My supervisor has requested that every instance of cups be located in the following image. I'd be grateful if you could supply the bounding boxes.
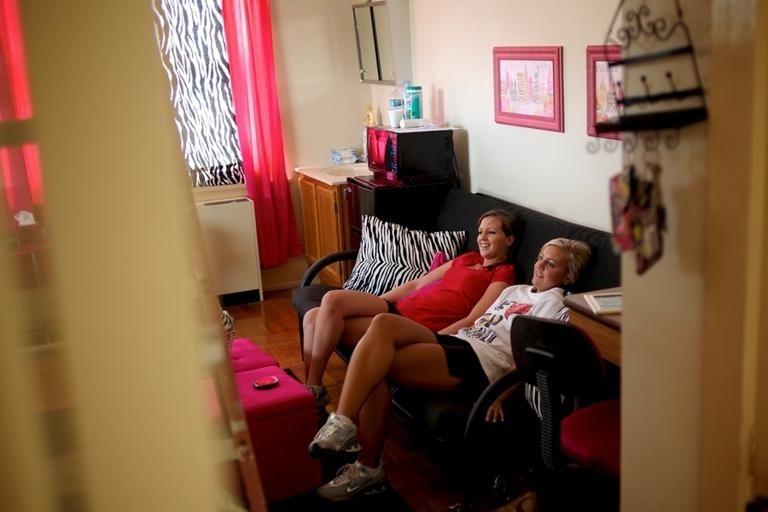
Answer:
[388,97,404,129]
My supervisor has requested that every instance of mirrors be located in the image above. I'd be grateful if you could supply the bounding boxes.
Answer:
[352,1,395,85]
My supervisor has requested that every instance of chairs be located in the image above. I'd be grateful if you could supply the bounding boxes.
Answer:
[511,314,620,484]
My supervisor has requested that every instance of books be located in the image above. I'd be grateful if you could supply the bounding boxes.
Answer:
[583,293,621,316]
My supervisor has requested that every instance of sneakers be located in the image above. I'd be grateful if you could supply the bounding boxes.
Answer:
[308,412,362,458]
[305,383,331,411]
[316,459,389,503]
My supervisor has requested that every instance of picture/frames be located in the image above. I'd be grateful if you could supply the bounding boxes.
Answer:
[586,44,625,140]
[494,46,564,133]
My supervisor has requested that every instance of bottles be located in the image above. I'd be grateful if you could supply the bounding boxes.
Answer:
[405,86,423,128]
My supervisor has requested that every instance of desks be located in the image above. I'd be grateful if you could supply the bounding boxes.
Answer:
[563,282,621,366]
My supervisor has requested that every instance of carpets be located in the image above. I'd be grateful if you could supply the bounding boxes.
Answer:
[265,367,416,512]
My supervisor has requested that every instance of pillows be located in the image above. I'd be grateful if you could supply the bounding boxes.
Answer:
[341,213,468,296]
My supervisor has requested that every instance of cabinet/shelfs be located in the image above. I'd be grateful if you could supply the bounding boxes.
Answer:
[297,173,351,289]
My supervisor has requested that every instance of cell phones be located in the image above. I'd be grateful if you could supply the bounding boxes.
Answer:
[253,375,278,388]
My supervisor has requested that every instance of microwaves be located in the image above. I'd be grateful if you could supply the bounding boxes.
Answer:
[365,126,453,183]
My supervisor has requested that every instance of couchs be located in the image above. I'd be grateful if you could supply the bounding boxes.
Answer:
[289,187,621,512]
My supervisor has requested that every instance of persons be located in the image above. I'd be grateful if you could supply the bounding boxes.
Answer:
[304,238,592,501]
[304,207,514,430]
